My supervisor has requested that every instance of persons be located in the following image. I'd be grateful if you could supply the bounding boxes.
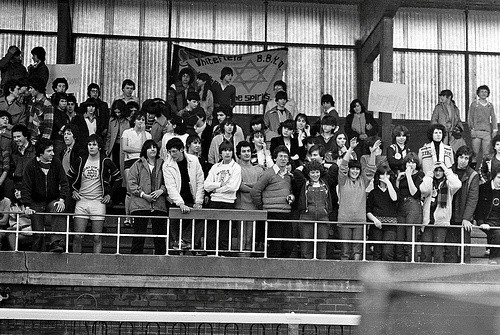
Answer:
[293,157,337,259]
[205,141,242,257]
[162,137,204,251]
[251,145,294,259]
[0,45,500,265]
[127,140,168,255]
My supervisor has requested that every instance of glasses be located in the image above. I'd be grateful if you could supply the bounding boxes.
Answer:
[91,90,100,94]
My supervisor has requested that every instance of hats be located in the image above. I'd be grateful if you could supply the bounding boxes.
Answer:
[439,90,452,95]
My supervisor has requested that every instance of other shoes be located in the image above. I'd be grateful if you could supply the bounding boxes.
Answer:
[50,245,64,253]
[488,260,498,265]
[123,219,131,226]
